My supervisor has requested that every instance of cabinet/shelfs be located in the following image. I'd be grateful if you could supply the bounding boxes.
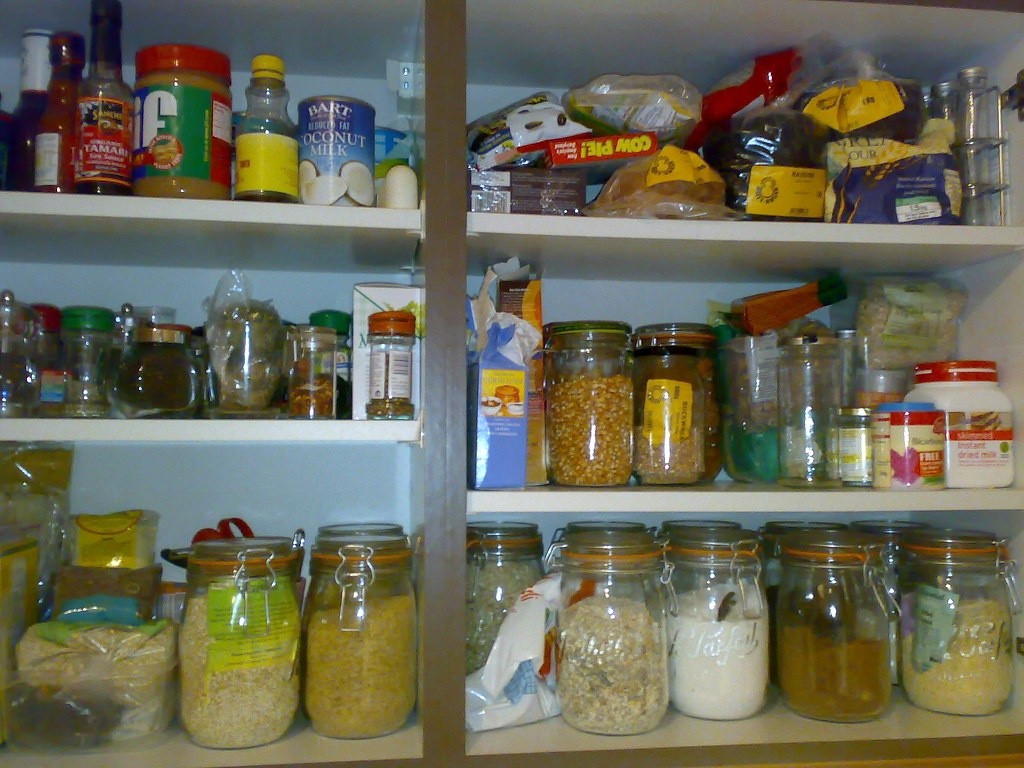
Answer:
[467,207,1024,768]
[2,187,426,768]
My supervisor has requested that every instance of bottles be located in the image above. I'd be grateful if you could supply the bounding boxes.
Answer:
[235,54,299,203]
[776,334,847,488]
[0,0,133,198]
[0,289,416,419]
[178,517,1015,747]
[543,323,723,502]
[923,67,994,227]
